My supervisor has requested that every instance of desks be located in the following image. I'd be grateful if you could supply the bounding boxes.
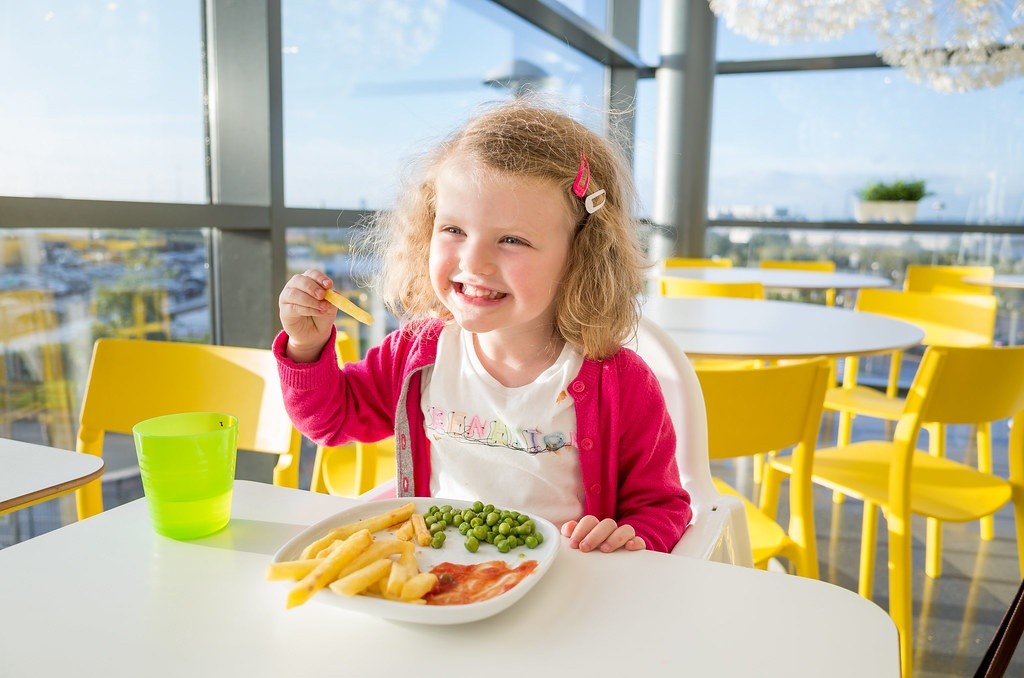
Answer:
[964,274,1024,289]
[646,267,893,289]
[631,295,924,361]
[0,438,903,678]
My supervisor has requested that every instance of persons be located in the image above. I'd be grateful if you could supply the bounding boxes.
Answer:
[273,108,692,554]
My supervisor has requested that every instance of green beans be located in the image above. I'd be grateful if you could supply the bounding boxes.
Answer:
[422,501,544,552]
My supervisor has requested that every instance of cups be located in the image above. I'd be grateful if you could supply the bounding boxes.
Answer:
[132,412,237,540]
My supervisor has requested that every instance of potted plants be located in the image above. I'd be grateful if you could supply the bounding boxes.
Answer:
[849,179,927,225]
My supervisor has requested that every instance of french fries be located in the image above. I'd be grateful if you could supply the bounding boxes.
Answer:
[324,288,374,326]
[267,500,439,610]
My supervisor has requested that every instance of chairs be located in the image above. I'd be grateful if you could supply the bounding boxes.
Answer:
[75,262,1024,678]
[0,234,379,527]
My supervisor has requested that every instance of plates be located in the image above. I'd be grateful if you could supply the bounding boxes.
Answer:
[269,496,561,624]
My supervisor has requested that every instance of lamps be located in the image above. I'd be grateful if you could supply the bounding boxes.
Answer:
[706,0,1024,97]
